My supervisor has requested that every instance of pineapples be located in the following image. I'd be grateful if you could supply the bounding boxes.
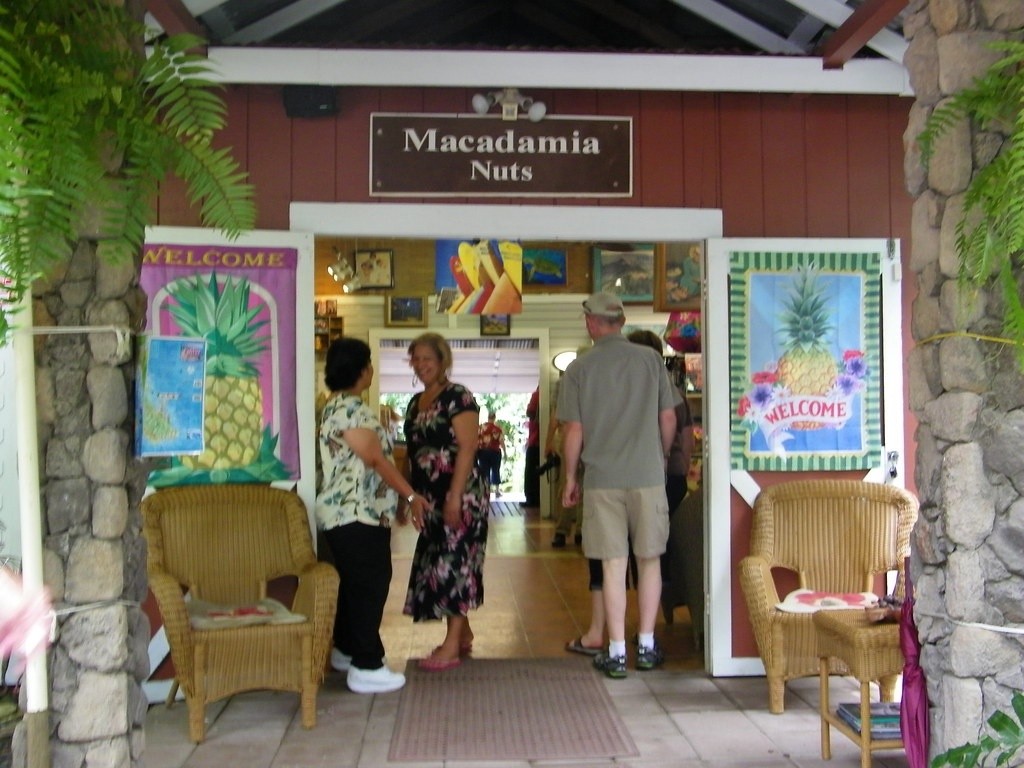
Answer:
[774,271,840,431]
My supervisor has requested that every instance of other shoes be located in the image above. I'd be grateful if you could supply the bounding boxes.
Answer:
[552,533,566,547]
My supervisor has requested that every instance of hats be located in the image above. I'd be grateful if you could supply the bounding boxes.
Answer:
[581,292,623,317]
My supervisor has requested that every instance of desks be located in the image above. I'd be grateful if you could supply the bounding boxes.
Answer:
[815,610,905,768]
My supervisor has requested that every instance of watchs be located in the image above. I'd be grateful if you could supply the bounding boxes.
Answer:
[407,491,418,504]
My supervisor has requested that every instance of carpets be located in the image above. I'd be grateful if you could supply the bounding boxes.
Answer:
[386,654,638,763]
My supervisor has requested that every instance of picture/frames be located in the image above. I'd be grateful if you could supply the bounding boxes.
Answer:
[351,249,393,288]
[524,247,568,284]
[480,314,509,335]
[590,243,702,311]
[386,291,428,325]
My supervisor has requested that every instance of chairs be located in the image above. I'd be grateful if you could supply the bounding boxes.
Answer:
[741,481,918,707]
[141,487,340,741]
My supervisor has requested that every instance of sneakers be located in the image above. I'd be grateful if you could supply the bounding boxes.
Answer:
[347,664,406,693]
[330,647,386,671]
[635,644,664,668]
[594,652,627,678]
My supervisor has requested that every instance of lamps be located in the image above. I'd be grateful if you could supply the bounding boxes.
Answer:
[471,86,548,123]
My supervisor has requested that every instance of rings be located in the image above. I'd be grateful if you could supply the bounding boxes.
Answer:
[412,515,416,523]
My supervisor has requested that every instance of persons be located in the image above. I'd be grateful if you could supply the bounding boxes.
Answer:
[357,253,388,285]
[474,411,507,499]
[565,527,639,653]
[390,332,489,673]
[554,290,684,679]
[551,345,589,549]
[519,381,540,508]
[317,337,433,695]
[626,329,695,646]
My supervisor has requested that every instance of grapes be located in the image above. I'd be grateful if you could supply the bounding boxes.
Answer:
[877,595,905,607]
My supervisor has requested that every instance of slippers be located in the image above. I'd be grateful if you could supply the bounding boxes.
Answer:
[433,631,473,651]
[419,647,461,671]
[566,637,603,655]
